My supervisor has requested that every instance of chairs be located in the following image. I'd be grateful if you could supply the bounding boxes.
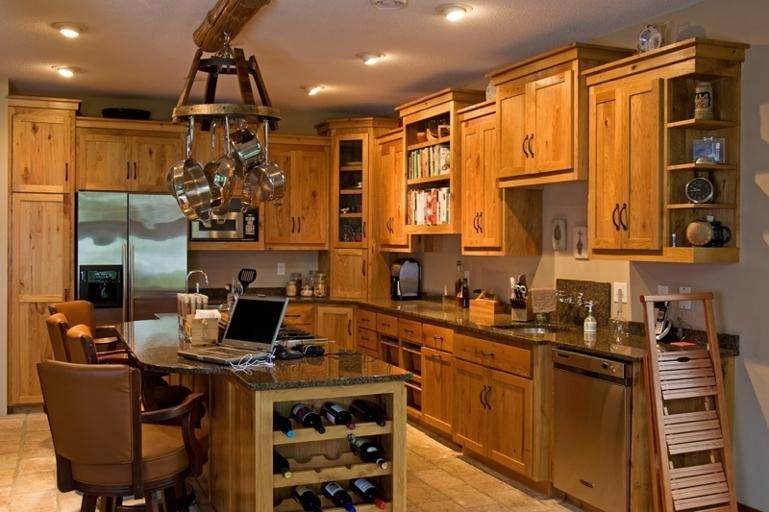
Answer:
[35,358,212,511]
[46,313,126,362]
[66,323,194,511]
[46,301,123,346]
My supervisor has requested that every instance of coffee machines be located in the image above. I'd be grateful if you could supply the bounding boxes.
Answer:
[389,256,422,300]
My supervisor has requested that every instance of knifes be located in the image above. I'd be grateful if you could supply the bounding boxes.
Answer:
[509,272,528,310]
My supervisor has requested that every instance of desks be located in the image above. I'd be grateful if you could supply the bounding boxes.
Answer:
[112,310,357,512]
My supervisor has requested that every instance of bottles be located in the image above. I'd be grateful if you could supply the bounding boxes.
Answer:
[274,400,388,511]
[176,293,210,319]
[612,287,629,352]
[454,259,470,308]
[694,80,713,121]
[286,270,328,298]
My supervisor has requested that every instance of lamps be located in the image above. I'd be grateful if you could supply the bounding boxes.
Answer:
[443,7,465,22]
[305,84,322,96]
[361,54,380,65]
[57,67,80,79]
[59,26,80,39]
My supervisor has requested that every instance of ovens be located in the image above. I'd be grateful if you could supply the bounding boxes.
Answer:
[549,347,631,511]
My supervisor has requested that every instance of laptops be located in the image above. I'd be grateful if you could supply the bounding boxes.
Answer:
[177,293,289,365]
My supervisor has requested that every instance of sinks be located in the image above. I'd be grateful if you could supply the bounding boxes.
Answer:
[488,322,573,335]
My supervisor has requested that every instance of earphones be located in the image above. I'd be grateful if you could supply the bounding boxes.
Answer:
[268,364,273,365]
[264,362,268,364]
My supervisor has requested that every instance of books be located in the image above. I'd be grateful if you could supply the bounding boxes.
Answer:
[406,145,450,225]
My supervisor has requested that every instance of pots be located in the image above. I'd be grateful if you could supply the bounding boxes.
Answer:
[159,114,286,221]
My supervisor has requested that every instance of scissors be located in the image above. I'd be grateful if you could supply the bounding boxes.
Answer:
[515,285,526,298]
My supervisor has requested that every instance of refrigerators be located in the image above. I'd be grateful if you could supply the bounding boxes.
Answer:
[76,191,189,324]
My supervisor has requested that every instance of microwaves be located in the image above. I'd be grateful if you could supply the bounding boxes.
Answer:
[189,207,259,240]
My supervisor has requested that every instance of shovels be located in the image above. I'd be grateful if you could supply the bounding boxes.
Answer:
[238,269,256,295]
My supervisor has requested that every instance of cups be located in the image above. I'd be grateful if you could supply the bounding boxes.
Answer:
[703,220,733,247]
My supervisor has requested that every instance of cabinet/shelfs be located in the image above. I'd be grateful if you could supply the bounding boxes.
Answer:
[376,313,421,413]
[10,96,81,193]
[394,82,485,235]
[452,331,551,480]
[282,304,315,334]
[456,99,542,258]
[374,128,426,253]
[10,194,72,405]
[330,249,397,299]
[225,381,408,512]
[354,308,376,355]
[315,305,354,348]
[331,116,375,248]
[262,135,328,250]
[421,323,452,425]
[591,35,750,264]
[76,117,183,192]
[496,42,634,187]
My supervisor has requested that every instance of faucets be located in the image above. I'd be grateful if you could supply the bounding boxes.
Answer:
[185,269,209,293]
[552,290,573,304]
